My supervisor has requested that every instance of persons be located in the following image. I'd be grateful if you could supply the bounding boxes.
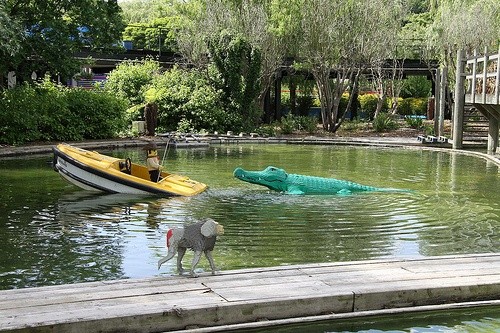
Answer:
[143,148,165,183]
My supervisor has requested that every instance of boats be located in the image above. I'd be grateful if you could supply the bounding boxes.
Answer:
[52,141,211,196]
